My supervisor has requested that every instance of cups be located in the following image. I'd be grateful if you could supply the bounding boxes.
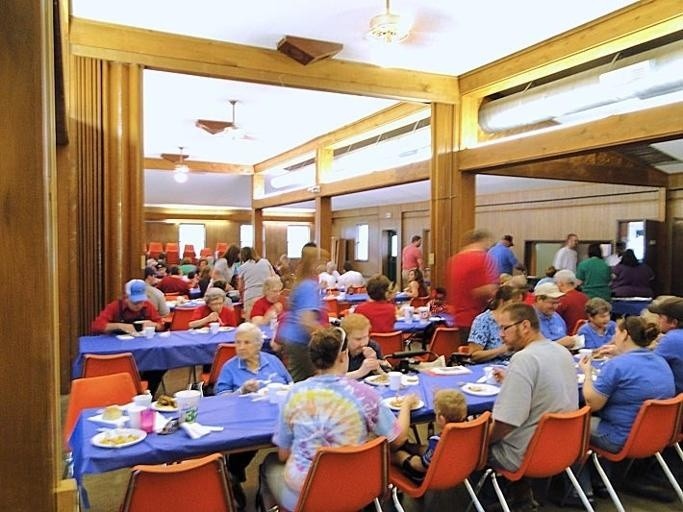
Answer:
[276,390,289,412]
[266,381,282,404]
[133,394,153,409]
[385,371,403,390]
[128,406,147,429]
[174,389,200,426]
[141,408,155,433]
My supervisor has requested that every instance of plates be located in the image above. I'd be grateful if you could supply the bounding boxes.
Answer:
[573,352,610,360]
[87,413,130,426]
[382,395,426,411]
[129,314,446,337]
[88,427,148,448]
[465,382,500,396]
[152,399,181,412]
[364,373,391,385]
[577,372,597,383]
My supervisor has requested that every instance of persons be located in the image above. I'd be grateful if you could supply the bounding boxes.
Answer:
[213,322,294,508]
[144,234,675,381]
[260,327,417,512]
[87,278,168,402]
[560,313,675,509]
[647,296,682,395]
[393,389,467,478]
[472,302,579,512]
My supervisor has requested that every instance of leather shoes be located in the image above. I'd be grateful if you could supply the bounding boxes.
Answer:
[548,494,595,509]
[595,484,609,498]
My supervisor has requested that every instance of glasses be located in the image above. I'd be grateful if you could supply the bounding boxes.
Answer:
[623,313,640,335]
[499,321,524,334]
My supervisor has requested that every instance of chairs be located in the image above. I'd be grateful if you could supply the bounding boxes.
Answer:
[573,321,587,337]
[322,286,461,368]
[63,373,139,512]
[650,392,682,469]
[62,242,292,398]
[495,406,595,512]
[120,452,235,512]
[592,394,682,512]
[388,411,511,512]
[278,436,390,512]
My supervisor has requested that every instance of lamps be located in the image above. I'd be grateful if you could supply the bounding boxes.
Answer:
[369,13,410,44]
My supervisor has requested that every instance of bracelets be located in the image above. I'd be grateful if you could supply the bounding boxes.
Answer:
[237,387,243,395]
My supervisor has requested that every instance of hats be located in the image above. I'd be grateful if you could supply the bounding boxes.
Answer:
[534,282,566,299]
[648,295,683,319]
[125,279,150,303]
